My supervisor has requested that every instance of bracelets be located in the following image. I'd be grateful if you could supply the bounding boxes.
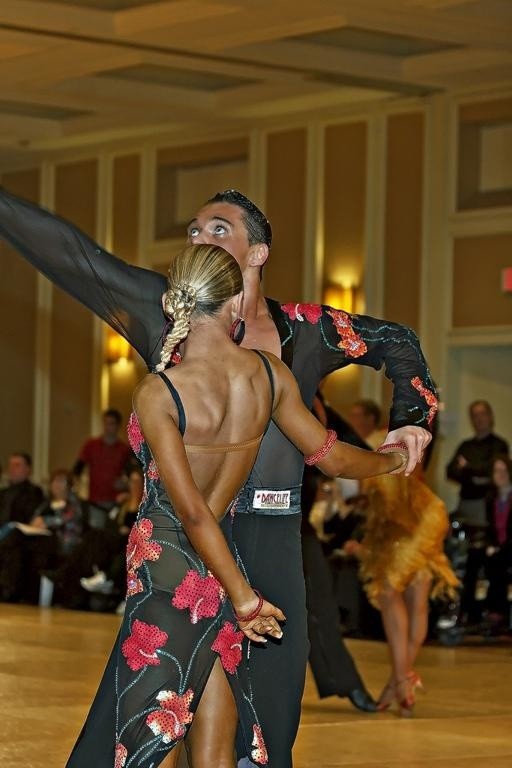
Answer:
[232,589,263,621]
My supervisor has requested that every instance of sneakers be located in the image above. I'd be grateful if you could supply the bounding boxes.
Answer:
[79,571,113,595]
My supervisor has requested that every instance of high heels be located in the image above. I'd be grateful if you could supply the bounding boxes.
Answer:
[375,670,424,716]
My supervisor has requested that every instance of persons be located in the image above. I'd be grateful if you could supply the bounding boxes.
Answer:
[1,189,440,768]
[63,244,410,768]
[312,454,511,642]
[2,452,144,615]
[320,399,382,558]
[73,409,133,506]
[446,402,510,521]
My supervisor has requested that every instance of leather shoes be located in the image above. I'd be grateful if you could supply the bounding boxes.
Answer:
[348,679,379,712]
